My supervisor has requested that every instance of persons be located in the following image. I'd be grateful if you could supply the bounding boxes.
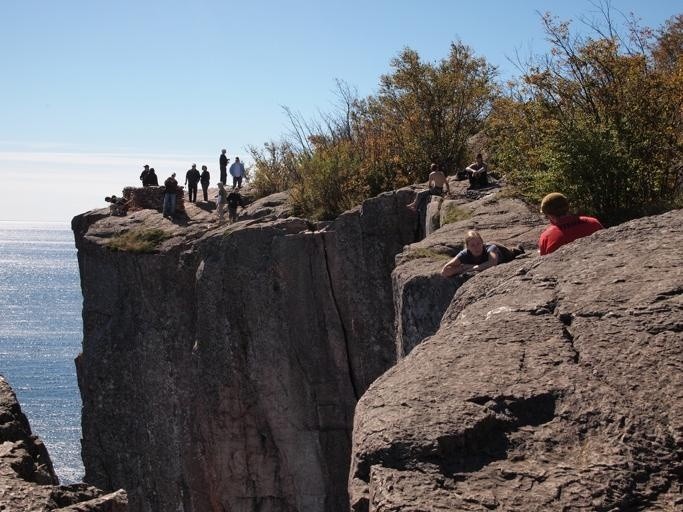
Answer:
[537,192,605,254]
[406,163,450,211]
[439,228,527,278]
[105,148,246,223]
[465,153,489,190]
